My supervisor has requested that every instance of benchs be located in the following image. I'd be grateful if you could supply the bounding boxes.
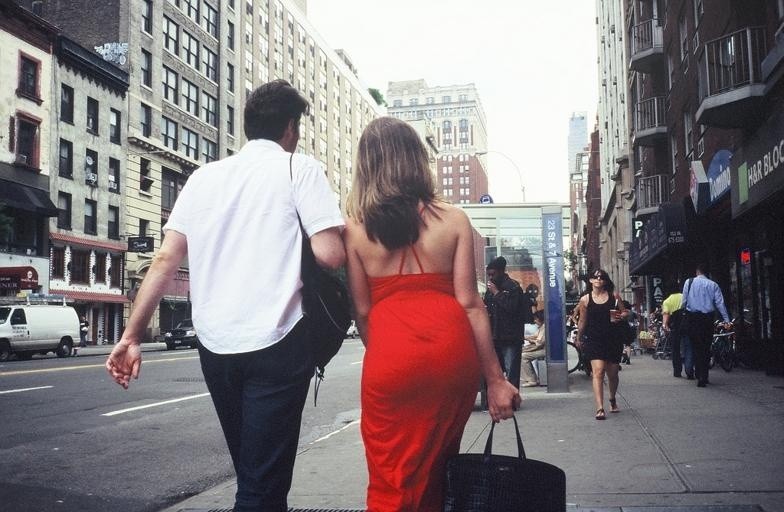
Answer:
[537,360,549,386]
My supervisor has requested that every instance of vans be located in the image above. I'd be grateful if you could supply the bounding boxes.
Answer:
[0,305,81,362]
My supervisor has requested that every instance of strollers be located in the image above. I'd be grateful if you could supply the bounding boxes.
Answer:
[652,322,672,360]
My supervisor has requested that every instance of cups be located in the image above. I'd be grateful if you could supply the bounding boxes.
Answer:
[608,309,619,323]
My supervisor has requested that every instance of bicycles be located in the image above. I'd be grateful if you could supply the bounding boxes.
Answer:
[712,305,765,373]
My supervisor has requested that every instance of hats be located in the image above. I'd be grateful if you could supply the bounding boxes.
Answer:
[486,256,506,271]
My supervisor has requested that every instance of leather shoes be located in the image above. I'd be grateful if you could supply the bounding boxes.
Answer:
[522,381,538,387]
[697,379,705,387]
[704,378,709,383]
[687,372,694,379]
[673,370,683,377]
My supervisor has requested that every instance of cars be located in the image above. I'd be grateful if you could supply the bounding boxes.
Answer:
[345,320,358,338]
[164,319,198,350]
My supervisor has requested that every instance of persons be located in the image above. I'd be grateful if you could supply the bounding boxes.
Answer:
[80,316,89,347]
[661,287,694,379]
[682,265,733,387]
[347,116,522,512]
[521,310,546,388]
[483,257,522,409]
[617,300,635,365]
[105,79,346,511]
[576,270,631,419]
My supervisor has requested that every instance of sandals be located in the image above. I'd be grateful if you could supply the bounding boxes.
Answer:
[595,409,606,420]
[608,399,620,412]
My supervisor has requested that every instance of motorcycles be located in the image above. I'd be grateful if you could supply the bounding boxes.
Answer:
[574,310,596,375]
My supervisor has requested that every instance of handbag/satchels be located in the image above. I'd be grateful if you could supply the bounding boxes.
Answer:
[290,153,353,373]
[680,278,693,328]
[444,414,567,511]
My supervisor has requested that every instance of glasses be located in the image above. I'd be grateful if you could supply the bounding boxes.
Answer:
[591,275,607,280]
